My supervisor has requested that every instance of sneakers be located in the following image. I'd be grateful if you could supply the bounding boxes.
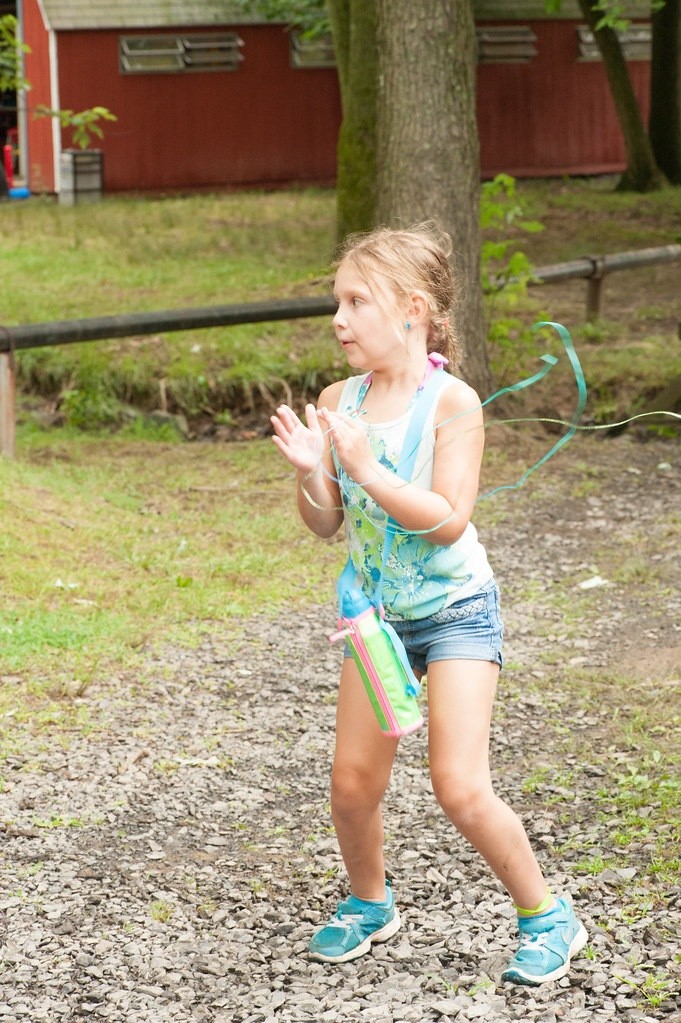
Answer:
[501,899,589,984]
[308,884,402,964]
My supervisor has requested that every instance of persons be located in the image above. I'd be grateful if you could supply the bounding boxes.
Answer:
[270,217,589,985]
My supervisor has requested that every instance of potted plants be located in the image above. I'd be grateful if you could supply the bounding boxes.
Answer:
[32,104,117,193]
